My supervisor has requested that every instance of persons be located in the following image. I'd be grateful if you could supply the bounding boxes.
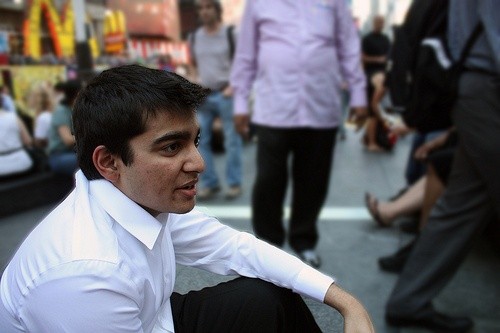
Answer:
[188,0,242,198]
[347,1,500,333]
[0,63,376,333]
[1,49,254,187]
[231,1,367,269]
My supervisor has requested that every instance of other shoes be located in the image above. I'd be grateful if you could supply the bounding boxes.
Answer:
[300,249,321,268]
[199,186,220,199]
[228,184,241,197]
[368,143,379,152]
[377,255,406,275]
[365,191,392,229]
